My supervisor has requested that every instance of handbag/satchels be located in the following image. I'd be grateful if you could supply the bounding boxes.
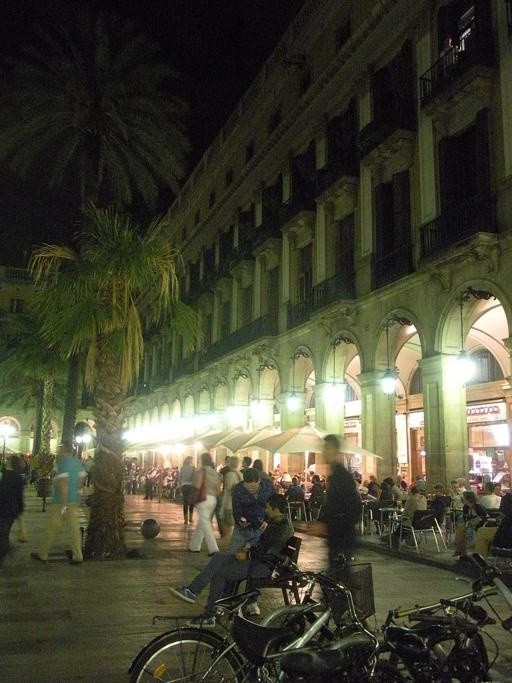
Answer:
[186,467,206,506]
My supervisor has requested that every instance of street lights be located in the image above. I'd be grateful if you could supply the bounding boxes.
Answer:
[3,421,12,467]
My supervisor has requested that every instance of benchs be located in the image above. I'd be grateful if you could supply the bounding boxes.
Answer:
[242,536,303,605]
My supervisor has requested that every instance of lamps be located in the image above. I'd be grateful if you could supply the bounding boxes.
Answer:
[286,294,479,417]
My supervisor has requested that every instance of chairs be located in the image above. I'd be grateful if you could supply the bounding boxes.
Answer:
[288,489,512,574]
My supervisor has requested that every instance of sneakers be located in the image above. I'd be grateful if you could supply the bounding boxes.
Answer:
[68,557,83,566]
[244,602,261,615]
[185,615,216,628]
[31,552,49,565]
[168,586,197,604]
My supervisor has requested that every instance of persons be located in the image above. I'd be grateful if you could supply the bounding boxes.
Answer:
[120,450,511,564]
[30,438,89,565]
[0,451,34,561]
[169,493,295,628]
[216,467,276,618]
[83,455,94,487]
[306,431,371,635]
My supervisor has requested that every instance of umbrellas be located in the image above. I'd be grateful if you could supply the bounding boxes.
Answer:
[184,421,385,462]
